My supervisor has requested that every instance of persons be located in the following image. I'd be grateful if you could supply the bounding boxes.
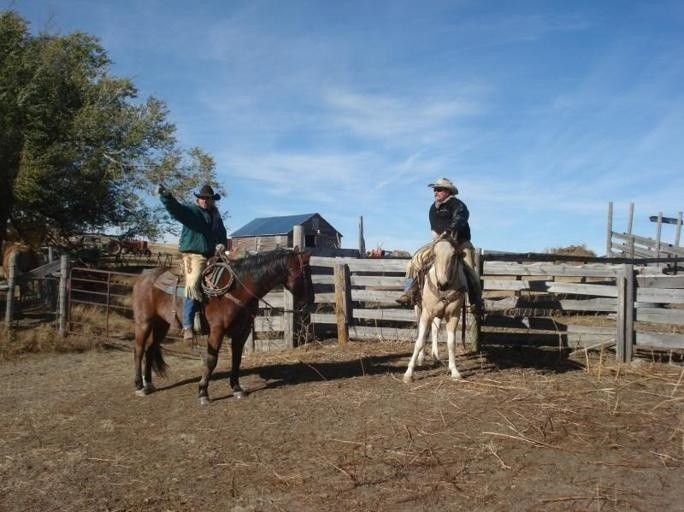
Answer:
[155,182,227,340]
[393,177,487,317]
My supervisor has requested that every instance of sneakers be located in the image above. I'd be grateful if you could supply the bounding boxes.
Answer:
[474,296,485,311]
[183,327,194,340]
[396,292,418,303]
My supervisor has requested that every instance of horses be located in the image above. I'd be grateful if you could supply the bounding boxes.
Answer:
[402,236,469,385]
[131,245,315,406]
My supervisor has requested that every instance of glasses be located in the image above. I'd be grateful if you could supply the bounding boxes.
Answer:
[433,187,444,192]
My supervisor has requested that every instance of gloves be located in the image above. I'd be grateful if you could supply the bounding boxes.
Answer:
[215,244,225,254]
[159,184,168,196]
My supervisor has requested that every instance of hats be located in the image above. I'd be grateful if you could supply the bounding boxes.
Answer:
[194,185,220,200]
[428,178,458,194]
